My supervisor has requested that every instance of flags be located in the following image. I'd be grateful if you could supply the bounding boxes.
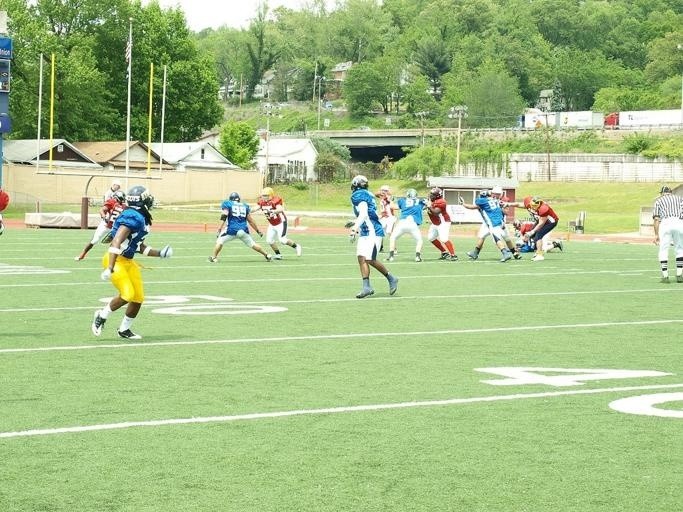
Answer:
[124,35,130,79]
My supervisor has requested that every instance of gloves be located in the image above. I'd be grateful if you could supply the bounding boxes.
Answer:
[458,196,464,206]
[258,231,263,239]
[160,245,172,258]
[264,210,274,220]
[349,229,359,244]
[525,230,535,237]
[422,199,431,209]
[216,229,221,238]
[101,267,111,281]
[499,201,511,208]
[345,220,355,229]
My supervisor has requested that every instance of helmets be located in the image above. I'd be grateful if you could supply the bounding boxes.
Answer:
[261,187,274,200]
[351,175,368,190]
[229,192,240,201]
[380,185,391,195]
[430,188,443,200]
[127,185,154,210]
[480,186,503,201]
[407,188,417,199]
[513,220,522,231]
[113,191,126,203]
[530,196,542,210]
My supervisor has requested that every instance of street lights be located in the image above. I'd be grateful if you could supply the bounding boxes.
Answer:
[413,111,430,147]
[446,104,469,177]
[260,102,284,186]
[316,75,327,130]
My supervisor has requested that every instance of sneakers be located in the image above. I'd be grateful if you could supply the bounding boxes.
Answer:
[501,253,522,262]
[208,256,217,263]
[92,310,106,335]
[415,253,422,261]
[356,289,374,299]
[389,275,398,295]
[530,252,544,262]
[659,277,670,283]
[440,252,458,261]
[553,240,563,251]
[466,251,479,260]
[676,275,683,283]
[296,243,302,256]
[265,253,282,262]
[74,256,89,261]
[116,328,141,341]
[383,249,398,262]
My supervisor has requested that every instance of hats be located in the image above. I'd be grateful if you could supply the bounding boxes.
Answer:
[112,179,121,185]
[659,185,672,193]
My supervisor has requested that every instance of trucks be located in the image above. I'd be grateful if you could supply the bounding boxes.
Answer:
[515,108,683,129]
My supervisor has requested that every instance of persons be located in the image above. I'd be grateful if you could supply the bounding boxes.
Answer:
[652,185,683,284]
[89,186,172,340]
[207,192,271,262]
[603,113,617,129]
[104,179,121,199]
[374,184,564,262]
[344,174,398,298]
[248,186,301,259]
[72,191,126,260]
